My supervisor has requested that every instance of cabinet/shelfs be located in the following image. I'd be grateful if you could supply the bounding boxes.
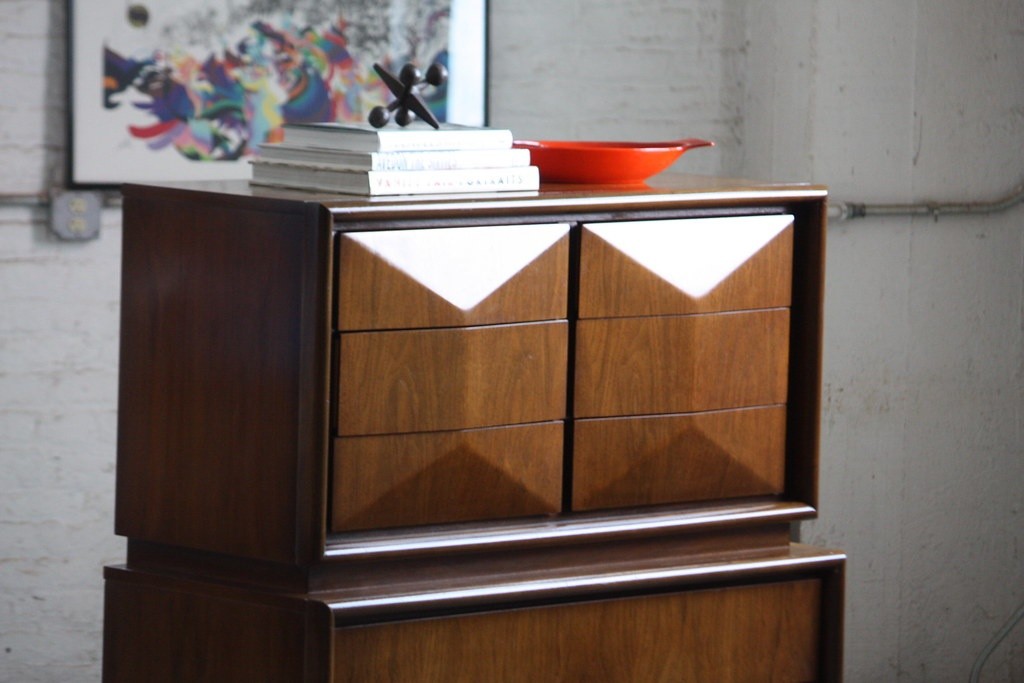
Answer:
[105,184,851,682]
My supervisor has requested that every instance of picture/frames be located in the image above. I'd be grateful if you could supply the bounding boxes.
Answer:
[67,1,493,189]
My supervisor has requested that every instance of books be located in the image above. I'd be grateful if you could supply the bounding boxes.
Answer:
[247,121,540,196]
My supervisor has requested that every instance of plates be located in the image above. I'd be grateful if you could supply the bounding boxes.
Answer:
[513,137,714,187]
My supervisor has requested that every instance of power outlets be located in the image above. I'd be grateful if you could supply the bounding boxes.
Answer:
[48,187,104,241]
